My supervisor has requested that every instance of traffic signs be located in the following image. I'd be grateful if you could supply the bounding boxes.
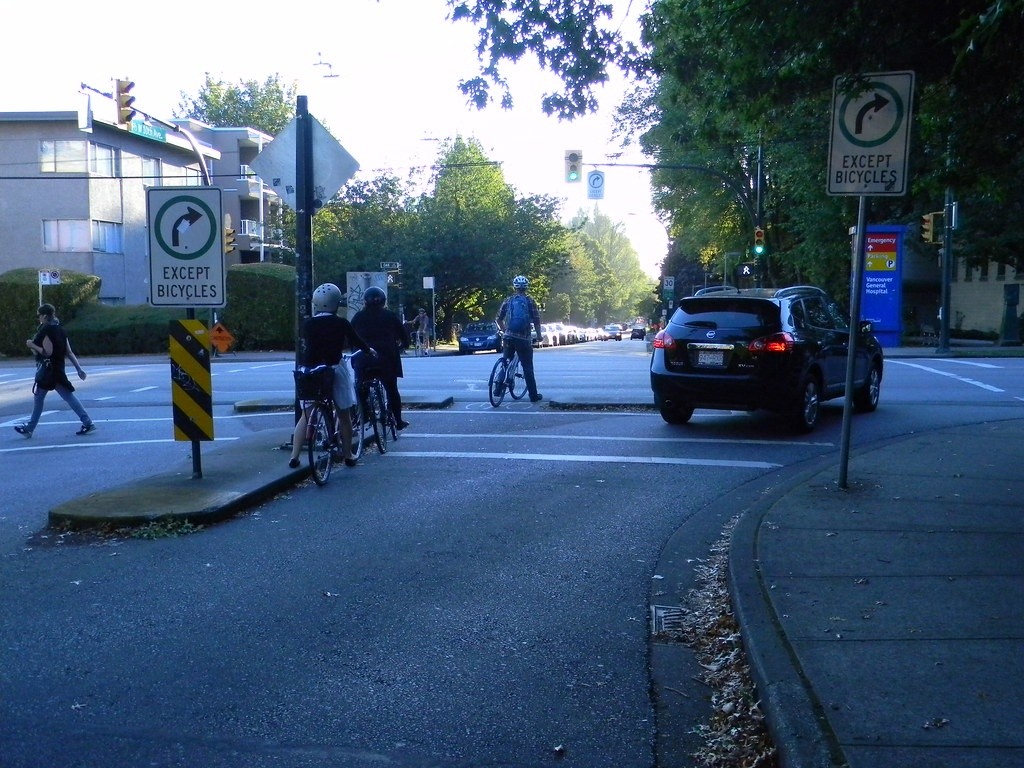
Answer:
[145,186,229,307]
[825,71,916,198]
[588,170,605,201]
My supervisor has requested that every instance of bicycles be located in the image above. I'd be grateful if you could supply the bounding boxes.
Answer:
[413,331,432,358]
[358,375,403,454]
[487,330,540,407]
[293,349,361,488]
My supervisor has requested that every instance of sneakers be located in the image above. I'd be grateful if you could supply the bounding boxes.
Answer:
[75,424,96,435]
[14,426,32,438]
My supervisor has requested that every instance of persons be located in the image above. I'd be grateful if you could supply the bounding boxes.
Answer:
[492,276,543,402]
[349,286,410,431]
[288,283,376,469]
[14,304,96,439]
[407,308,430,355]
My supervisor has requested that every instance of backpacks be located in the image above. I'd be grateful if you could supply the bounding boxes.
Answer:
[505,293,532,333]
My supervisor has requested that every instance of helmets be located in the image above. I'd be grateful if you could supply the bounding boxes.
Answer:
[418,308,425,313]
[513,276,529,288]
[313,283,341,311]
[363,286,386,308]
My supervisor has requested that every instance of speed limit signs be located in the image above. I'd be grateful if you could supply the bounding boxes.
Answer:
[664,277,674,290]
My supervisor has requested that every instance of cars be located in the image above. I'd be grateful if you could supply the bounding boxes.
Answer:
[603,325,622,341]
[459,321,503,356]
[530,322,604,348]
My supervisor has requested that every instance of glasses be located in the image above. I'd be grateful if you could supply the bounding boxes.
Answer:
[36,314,42,319]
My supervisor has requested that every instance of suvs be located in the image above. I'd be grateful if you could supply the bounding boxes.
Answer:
[650,286,884,434]
[630,329,644,341]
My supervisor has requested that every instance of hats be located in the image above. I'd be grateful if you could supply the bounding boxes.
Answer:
[37,305,55,315]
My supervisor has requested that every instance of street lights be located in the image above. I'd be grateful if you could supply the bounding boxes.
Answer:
[704,273,716,293]
[692,285,702,296]
[724,251,742,291]
[627,212,673,323]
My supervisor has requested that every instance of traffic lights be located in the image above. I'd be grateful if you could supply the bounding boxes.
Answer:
[922,211,938,244]
[735,264,754,276]
[565,149,582,183]
[225,226,237,256]
[114,79,137,128]
[754,229,765,255]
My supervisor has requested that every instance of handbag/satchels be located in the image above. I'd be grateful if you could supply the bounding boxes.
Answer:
[293,366,335,400]
[333,356,357,409]
[35,349,57,391]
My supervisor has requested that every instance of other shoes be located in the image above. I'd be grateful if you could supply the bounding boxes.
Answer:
[289,458,300,467]
[346,456,359,463]
[417,346,419,349]
[425,351,428,354]
[396,421,409,431]
[493,389,502,396]
[531,394,543,401]
